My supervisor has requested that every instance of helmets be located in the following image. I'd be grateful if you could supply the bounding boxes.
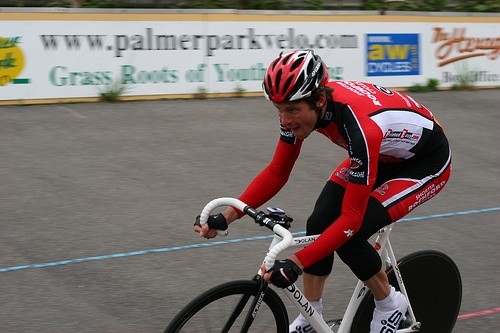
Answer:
[262,49,328,103]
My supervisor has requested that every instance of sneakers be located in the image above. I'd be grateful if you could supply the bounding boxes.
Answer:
[369,291,408,333]
[289,312,323,333]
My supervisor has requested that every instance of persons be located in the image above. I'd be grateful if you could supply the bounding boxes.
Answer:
[193,49,451,333]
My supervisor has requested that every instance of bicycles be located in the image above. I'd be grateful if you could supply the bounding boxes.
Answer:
[162,196,463,333]
